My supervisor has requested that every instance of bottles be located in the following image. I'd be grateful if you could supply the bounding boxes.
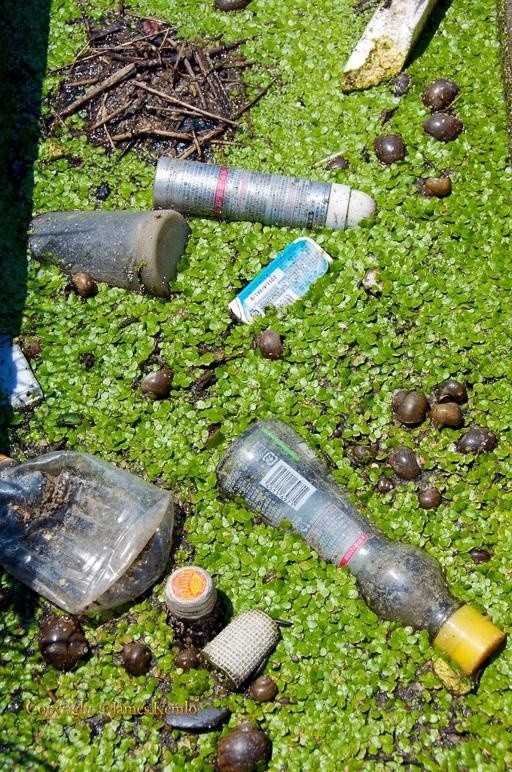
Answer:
[212,416,506,681]
